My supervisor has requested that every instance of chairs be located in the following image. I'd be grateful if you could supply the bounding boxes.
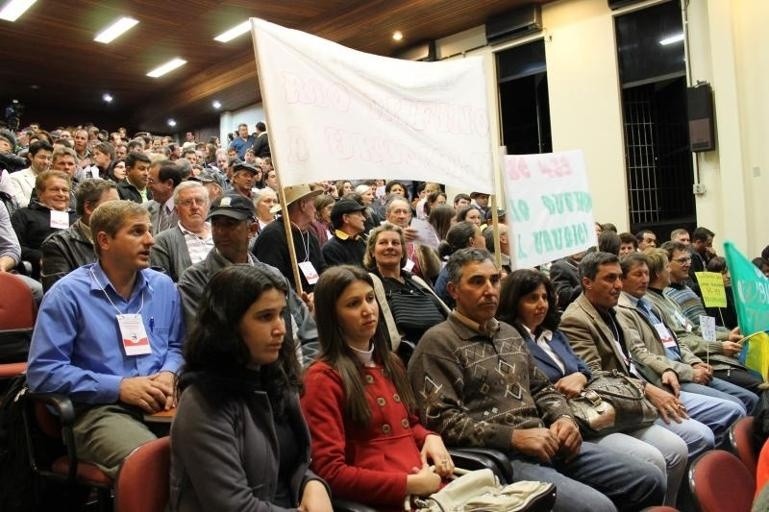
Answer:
[112,434,173,512]
[0,271,39,409]
[13,369,116,511]
[442,412,769,512]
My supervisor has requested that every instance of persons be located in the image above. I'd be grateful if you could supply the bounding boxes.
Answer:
[707,256,737,324]
[409,244,664,510]
[1,117,768,450]
[494,268,687,512]
[301,264,457,511]
[26,199,180,492]
[555,251,745,506]
[171,259,335,512]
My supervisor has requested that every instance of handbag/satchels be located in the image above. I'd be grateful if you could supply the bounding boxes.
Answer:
[417,469,555,511]
[567,368,658,442]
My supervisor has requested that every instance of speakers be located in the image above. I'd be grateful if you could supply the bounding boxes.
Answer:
[607,0,646,11]
[486,0,543,45]
[685,86,715,153]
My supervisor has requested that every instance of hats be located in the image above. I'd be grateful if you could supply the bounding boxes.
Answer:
[331,201,366,221]
[270,185,323,213]
[187,164,258,220]
